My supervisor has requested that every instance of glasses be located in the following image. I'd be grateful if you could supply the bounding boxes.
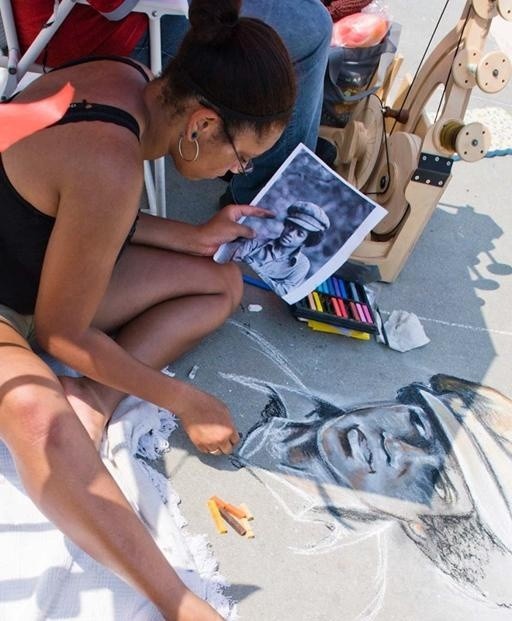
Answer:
[197,100,254,179]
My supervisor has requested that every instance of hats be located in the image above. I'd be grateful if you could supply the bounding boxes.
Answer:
[285,202,330,233]
[416,374,512,549]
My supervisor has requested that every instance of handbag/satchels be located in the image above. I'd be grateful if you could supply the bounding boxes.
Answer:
[319,28,392,129]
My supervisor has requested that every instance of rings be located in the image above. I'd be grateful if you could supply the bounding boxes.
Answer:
[208,448,220,456]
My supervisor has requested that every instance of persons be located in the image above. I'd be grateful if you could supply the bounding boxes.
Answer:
[218,317,511,621]
[233,202,330,296]
[10,3,339,215]
[1,14,300,621]
